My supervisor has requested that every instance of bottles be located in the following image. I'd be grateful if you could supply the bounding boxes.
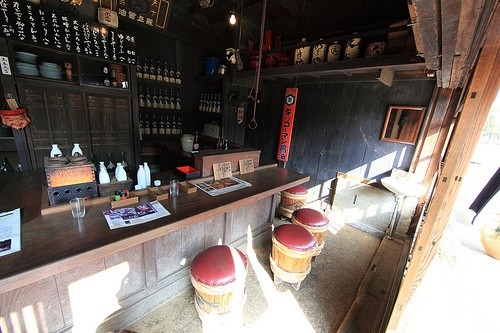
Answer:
[49,143,62,158]
[102,66,129,88]
[135,56,182,84]
[198,93,221,113]
[144,162,152,186]
[114,162,128,182]
[98,161,111,184]
[140,114,183,136]
[136,86,182,111]
[114,189,130,201]
[193,131,200,151]
[136,165,147,189]
[216,136,228,151]
[292,32,386,66]
[71,143,83,156]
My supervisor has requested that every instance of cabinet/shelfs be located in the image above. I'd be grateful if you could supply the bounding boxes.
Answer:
[0,37,135,173]
[135,54,184,165]
[194,56,227,151]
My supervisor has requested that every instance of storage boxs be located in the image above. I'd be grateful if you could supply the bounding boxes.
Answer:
[44,156,134,207]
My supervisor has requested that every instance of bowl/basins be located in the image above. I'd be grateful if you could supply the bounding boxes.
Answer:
[15,51,63,80]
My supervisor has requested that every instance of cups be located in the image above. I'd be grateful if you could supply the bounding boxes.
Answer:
[170,179,180,197]
[69,198,86,219]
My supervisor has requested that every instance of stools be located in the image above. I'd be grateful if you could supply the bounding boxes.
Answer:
[189,245,249,329]
[279,186,308,220]
[292,208,329,262]
[269,224,321,290]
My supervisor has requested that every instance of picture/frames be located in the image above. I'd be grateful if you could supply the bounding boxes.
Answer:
[380,104,427,145]
